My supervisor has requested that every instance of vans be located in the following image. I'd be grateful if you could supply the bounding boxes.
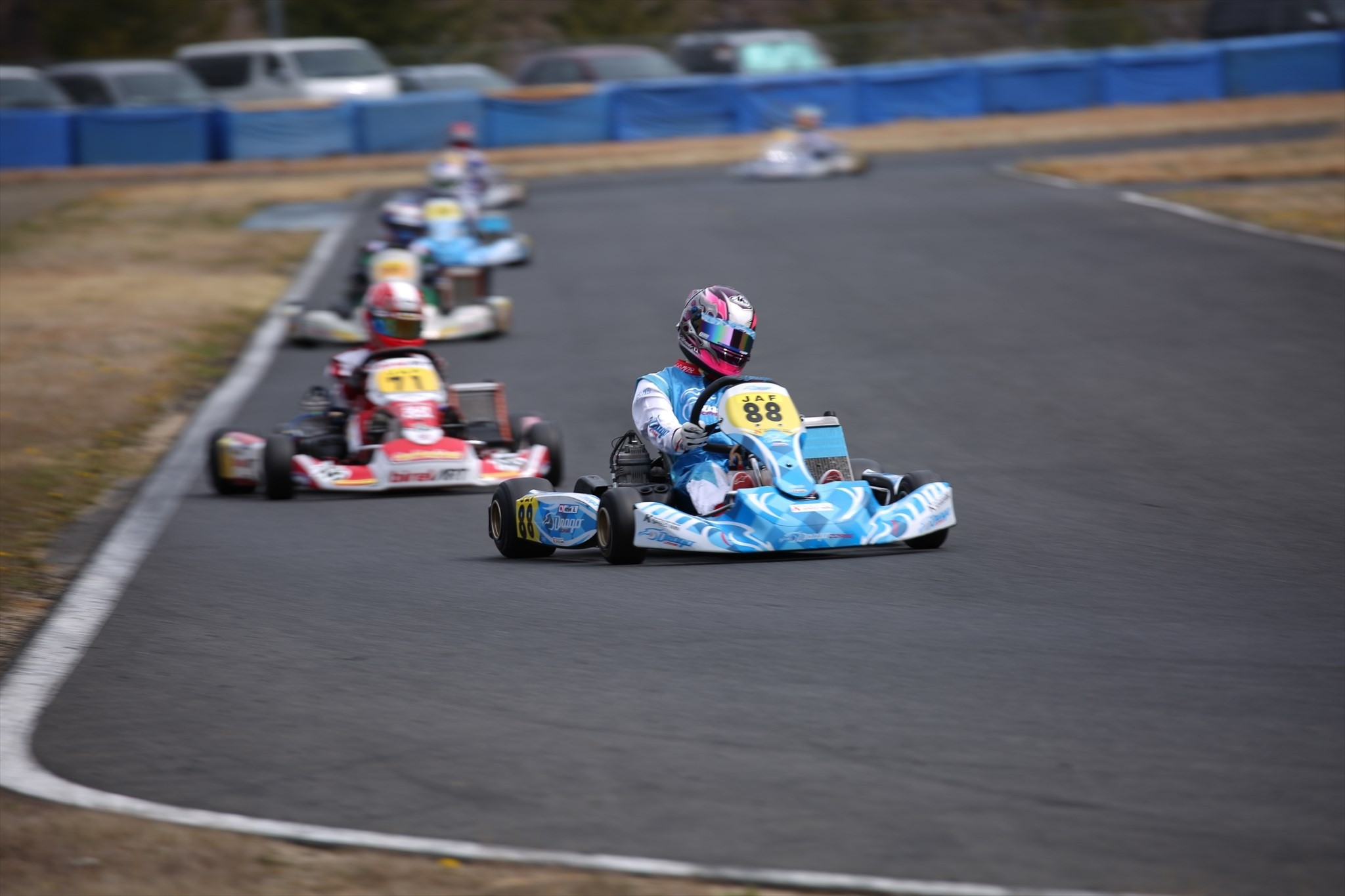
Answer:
[176,37,399,106]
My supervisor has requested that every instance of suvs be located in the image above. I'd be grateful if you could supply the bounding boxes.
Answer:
[676,28,836,77]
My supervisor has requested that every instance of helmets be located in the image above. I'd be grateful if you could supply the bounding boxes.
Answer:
[361,282,427,349]
[675,286,756,375]
[365,249,422,285]
[423,197,466,239]
[430,151,467,188]
[791,104,824,127]
[449,123,476,146]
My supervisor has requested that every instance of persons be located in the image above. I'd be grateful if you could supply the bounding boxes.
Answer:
[345,202,453,315]
[437,120,496,202]
[413,163,486,242]
[785,105,838,159]
[322,280,465,464]
[632,286,843,516]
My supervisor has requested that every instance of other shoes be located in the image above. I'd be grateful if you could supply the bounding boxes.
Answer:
[818,469,844,485]
[715,471,753,516]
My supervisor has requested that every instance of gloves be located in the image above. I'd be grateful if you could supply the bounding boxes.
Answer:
[342,369,367,399]
[671,420,708,454]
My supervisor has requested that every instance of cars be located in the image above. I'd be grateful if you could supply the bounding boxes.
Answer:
[506,44,689,85]
[42,59,231,110]
[1,64,72,111]
[396,62,516,97]
